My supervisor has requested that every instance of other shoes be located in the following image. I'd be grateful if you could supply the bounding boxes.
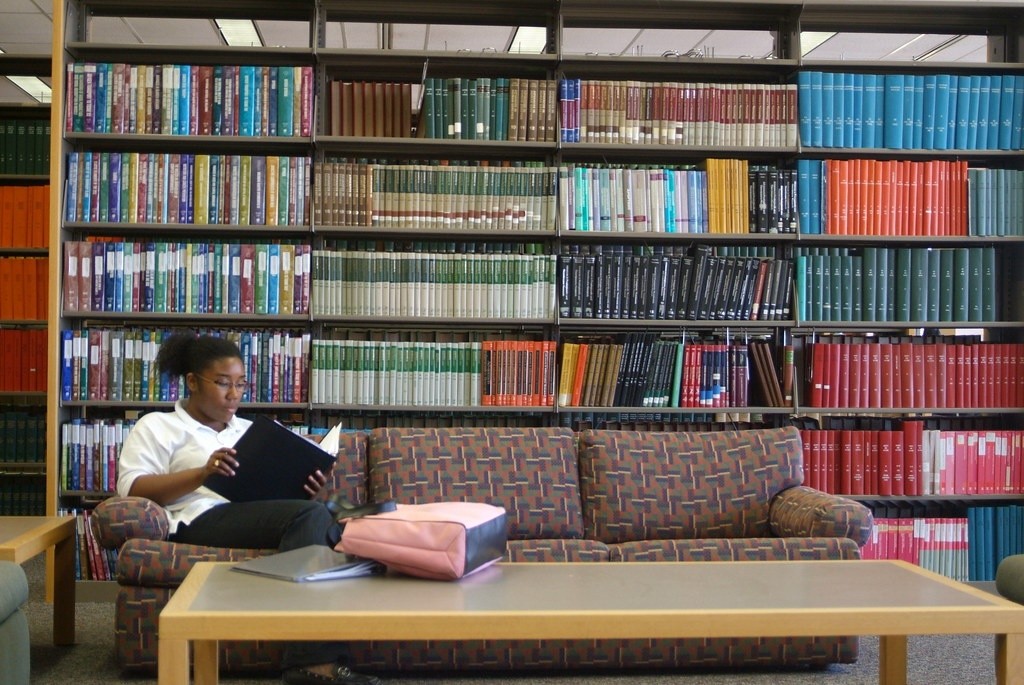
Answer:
[281,666,383,685]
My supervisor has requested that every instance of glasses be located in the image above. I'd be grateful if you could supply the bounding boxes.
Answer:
[195,373,249,392]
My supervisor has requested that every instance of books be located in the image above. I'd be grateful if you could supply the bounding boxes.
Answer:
[0,114,48,515]
[278,415,1023,497]
[232,544,384,581]
[201,421,342,503]
[59,418,137,494]
[67,155,1024,239]
[860,501,1024,580]
[68,62,1024,151]
[56,507,120,581]
[60,234,1000,321]
[53,323,1024,411]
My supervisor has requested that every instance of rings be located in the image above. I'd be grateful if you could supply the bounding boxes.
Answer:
[214,459,221,466]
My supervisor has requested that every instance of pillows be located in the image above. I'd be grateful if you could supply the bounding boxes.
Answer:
[368,426,581,538]
[578,425,804,543]
[302,432,369,523]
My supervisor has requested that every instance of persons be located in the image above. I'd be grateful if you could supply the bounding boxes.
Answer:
[116,331,341,554]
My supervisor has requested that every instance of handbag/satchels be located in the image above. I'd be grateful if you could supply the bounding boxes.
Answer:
[325,501,507,582]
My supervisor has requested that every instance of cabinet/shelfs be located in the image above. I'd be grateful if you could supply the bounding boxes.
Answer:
[46,0,1024,602]
[0,54,54,516]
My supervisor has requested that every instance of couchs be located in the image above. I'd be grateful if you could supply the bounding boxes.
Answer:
[90,427,874,679]
[0,560,30,685]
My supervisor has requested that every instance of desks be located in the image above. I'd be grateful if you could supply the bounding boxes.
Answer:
[0,516,78,649]
[159,559,1024,685]
